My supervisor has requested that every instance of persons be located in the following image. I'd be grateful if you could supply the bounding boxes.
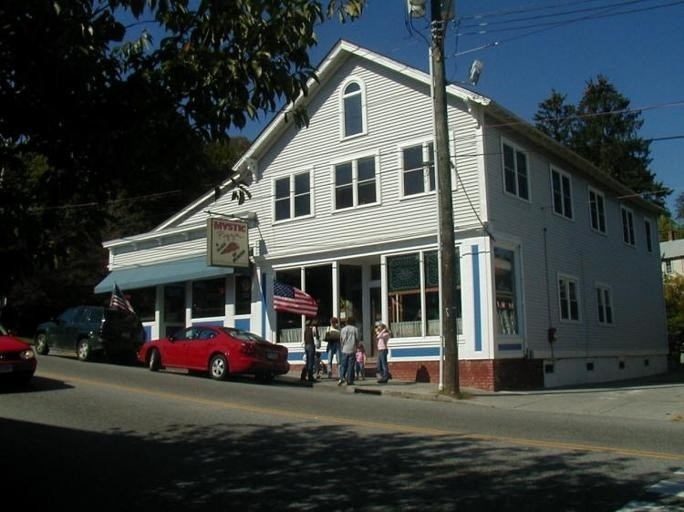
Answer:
[375,322,391,383]
[298,317,367,385]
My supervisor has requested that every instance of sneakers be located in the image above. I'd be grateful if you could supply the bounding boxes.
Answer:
[338,378,345,385]
[301,377,308,383]
[347,379,353,384]
[309,377,316,382]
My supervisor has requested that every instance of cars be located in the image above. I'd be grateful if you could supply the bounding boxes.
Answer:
[0,322,37,386]
[138,323,291,382]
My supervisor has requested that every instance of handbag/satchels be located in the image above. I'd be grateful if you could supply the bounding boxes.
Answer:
[325,331,340,341]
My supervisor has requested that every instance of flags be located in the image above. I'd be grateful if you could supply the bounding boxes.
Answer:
[272,277,319,318]
[109,282,133,311]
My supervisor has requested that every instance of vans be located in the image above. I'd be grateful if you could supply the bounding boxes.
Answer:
[35,304,146,360]
